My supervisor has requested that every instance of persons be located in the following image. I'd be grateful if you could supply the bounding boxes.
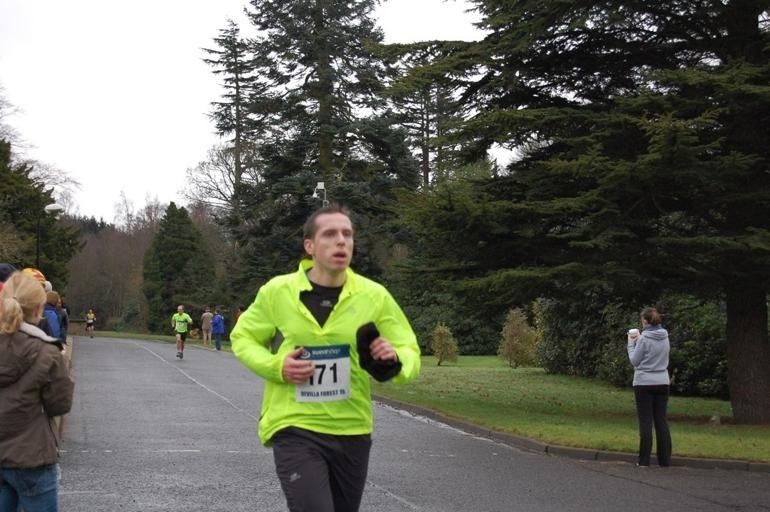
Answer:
[0,261,72,355]
[0,270,77,511]
[85,308,96,339]
[235,306,243,320]
[229,202,426,511]
[199,306,213,346]
[170,304,193,361]
[209,308,225,351]
[626,304,674,469]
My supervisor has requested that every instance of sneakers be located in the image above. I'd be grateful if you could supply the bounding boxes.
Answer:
[176,352,183,360]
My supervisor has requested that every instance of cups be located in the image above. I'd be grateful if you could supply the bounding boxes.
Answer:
[629,330,638,340]
